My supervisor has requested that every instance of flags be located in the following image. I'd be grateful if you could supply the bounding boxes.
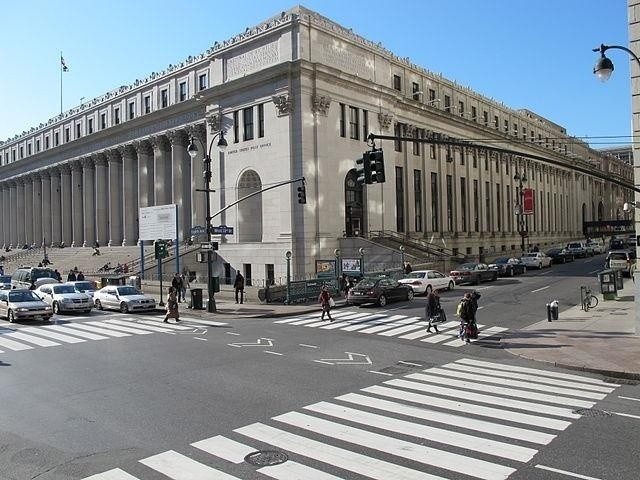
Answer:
[61,56,70,70]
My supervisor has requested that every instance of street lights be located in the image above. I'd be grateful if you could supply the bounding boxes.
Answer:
[399,245,405,268]
[590,41,640,84]
[513,157,528,256]
[184,129,232,314]
[284,251,293,305]
[359,247,368,274]
[333,248,341,296]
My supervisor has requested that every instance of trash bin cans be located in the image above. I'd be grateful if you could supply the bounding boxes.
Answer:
[190,289,202,309]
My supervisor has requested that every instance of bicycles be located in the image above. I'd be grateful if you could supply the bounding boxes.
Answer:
[580,285,598,313]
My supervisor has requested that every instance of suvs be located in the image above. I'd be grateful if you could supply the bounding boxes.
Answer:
[11,266,58,291]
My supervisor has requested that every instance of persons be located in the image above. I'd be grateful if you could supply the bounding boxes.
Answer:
[114,263,129,274]
[403,261,413,274]
[454,290,481,345]
[38,262,43,267]
[425,289,446,333]
[67,266,84,282]
[340,273,365,299]
[318,286,336,322]
[98,261,111,272]
[172,272,191,303]
[234,270,246,304]
[91,240,100,256]
[55,269,63,283]
[163,287,182,324]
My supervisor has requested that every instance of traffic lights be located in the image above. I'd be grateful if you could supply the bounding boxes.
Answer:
[356,153,373,184]
[297,186,306,204]
[370,151,386,183]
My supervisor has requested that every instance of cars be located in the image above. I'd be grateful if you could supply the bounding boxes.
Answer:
[347,276,415,306]
[449,262,498,286]
[0,275,11,289]
[0,290,53,325]
[32,277,94,315]
[488,256,527,277]
[94,285,156,314]
[68,281,94,295]
[398,269,456,297]
[521,234,636,275]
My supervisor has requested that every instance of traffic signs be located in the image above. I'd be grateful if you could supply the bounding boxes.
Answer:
[190,225,206,235]
[211,226,233,234]
